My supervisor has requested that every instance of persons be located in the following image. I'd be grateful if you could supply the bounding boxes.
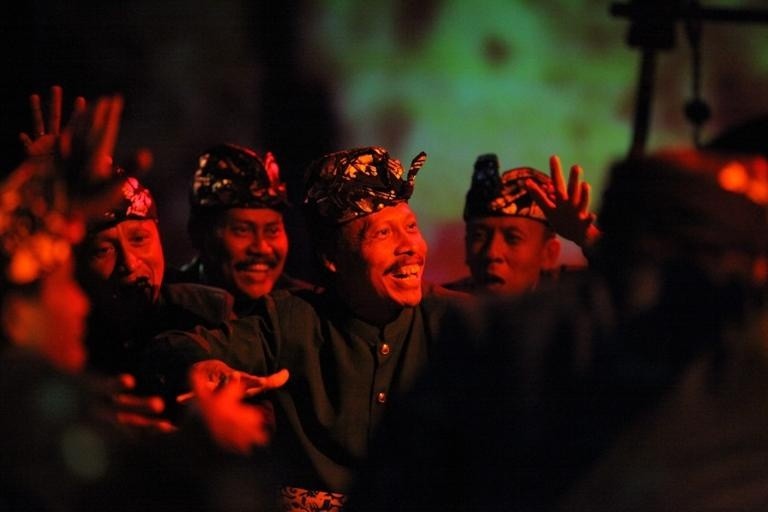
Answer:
[1,85,768,511]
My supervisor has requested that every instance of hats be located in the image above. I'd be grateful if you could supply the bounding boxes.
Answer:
[81,172,162,234]
[298,144,428,232]
[186,144,290,216]
[462,153,565,228]
